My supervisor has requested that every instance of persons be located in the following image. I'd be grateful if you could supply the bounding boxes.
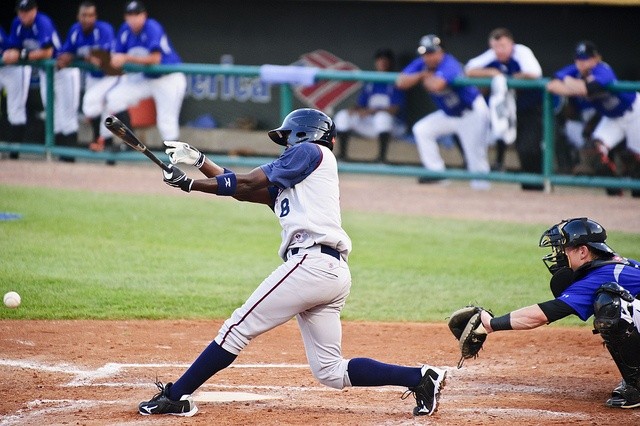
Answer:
[56,0,117,165]
[463,26,545,191]
[395,34,492,191]
[2,0,65,161]
[99,0,187,171]
[586,90,639,198]
[332,47,409,163]
[137,108,448,418]
[448,217,640,410]
[0,25,27,160]
[545,40,616,179]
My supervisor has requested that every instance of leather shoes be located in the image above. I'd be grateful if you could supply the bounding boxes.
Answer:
[162,160,195,193]
[163,140,206,169]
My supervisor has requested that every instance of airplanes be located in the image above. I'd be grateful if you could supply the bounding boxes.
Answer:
[447,306,494,360]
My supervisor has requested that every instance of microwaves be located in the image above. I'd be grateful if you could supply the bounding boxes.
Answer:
[104,116,171,173]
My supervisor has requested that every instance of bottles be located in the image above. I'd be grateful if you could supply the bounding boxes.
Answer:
[124,0,145,14]
[15,0,38,11]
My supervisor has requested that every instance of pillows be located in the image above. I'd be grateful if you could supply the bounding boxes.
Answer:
[572,40,599,60]
[267,108,336,151]
[539,217,616,276]
[414,34,442,56]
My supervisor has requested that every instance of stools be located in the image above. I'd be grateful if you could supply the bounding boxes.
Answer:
[282,243,340,263]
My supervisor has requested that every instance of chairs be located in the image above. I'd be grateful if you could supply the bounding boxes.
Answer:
[605,383,640,409]
[399,363,447,417]
[137,373,199,417]
[610,376,628,398]
